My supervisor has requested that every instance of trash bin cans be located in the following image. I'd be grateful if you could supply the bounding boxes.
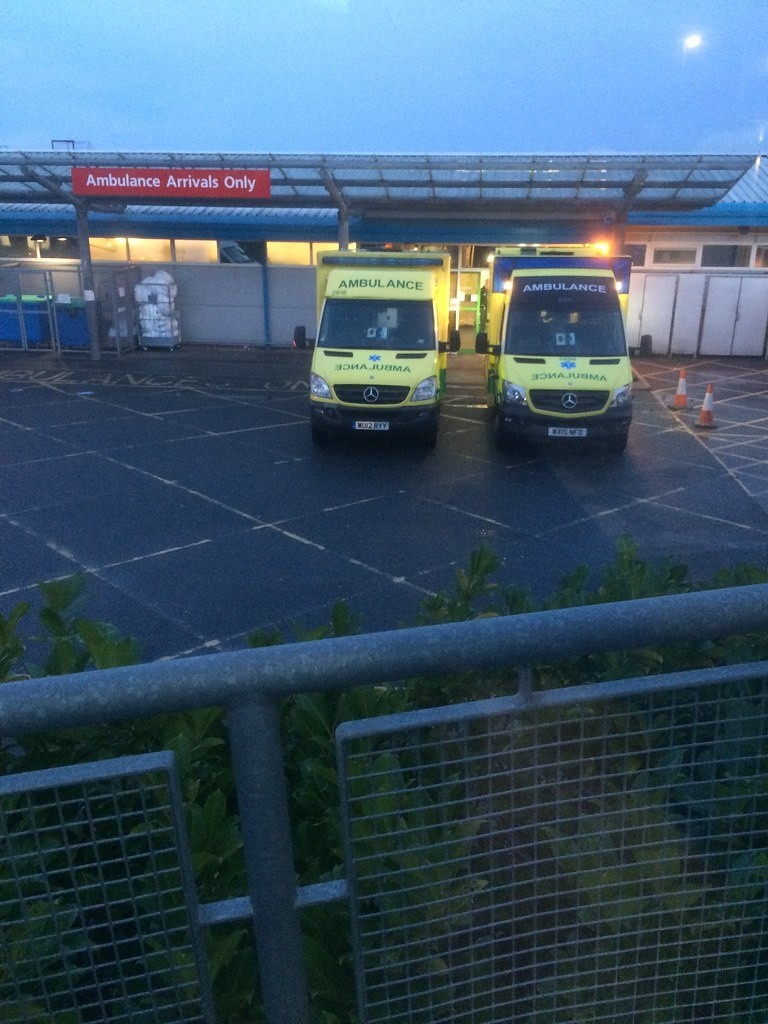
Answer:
[52,296,94,349]
[0,295,51,346]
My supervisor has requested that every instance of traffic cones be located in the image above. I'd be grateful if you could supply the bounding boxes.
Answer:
[665,363,692,410]
[693,383,719,429]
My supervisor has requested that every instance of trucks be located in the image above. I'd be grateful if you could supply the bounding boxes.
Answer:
[290,250,460,448]
[475,251,653,459]
